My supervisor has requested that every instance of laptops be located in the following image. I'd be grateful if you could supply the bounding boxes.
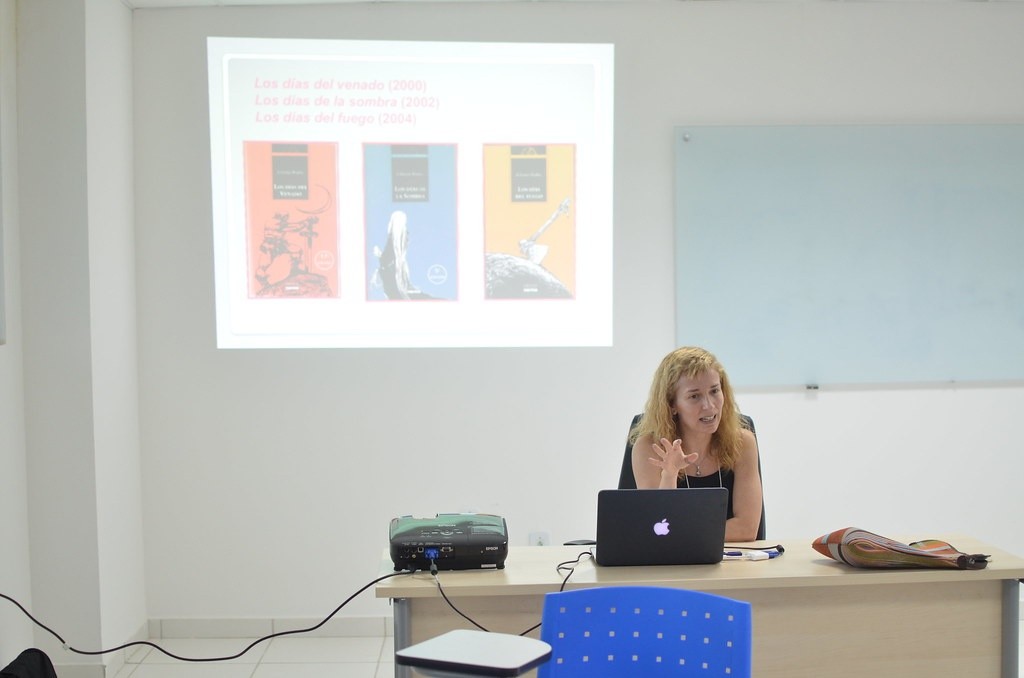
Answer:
[591,487,729,567]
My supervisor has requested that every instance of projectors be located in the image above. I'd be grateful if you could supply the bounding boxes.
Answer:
[389,513,509,570]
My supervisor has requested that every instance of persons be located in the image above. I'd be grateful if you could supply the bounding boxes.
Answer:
[629,347,763,542]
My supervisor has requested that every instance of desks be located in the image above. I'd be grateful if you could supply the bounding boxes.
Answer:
[376,536,1024,678]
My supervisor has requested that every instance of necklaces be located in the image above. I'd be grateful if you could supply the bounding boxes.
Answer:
[692,456,707,476]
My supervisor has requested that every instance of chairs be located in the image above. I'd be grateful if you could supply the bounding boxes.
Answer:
[537,585,752,677]
